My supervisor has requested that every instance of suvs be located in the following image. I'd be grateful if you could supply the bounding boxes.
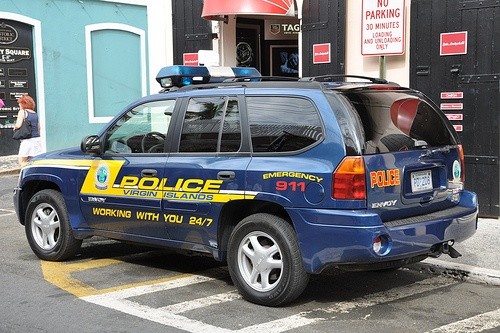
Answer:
[12,62,481,313]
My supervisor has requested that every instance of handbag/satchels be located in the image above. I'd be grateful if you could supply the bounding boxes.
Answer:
[12,110,32,141]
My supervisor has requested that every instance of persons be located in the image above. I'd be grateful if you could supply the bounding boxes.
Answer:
[13,95,40,166]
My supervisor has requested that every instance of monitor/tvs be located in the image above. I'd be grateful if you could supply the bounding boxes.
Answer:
[270,45,299,77]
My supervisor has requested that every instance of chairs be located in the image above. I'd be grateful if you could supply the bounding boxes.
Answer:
[183,119,210,149]
[208,119,230,133]
